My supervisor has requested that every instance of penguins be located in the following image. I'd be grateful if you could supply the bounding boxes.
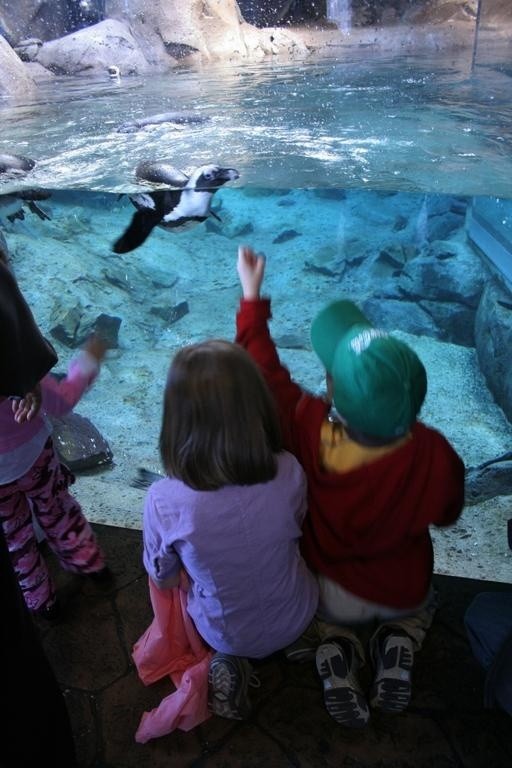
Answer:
[107,66,121,84]
[0,152,51,222]
[112,160,240,254]
[117,111,210,134]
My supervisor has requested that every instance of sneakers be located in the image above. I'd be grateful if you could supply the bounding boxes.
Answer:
[316,628,370,728]
[72,567,114,590]
[283,635,320,662]
[369,629,414,713]
[208,652,253,720]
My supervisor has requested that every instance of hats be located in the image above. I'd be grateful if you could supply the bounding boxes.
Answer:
[312,301,428,435]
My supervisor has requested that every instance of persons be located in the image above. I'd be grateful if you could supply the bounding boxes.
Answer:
[234,245,466,727]
[143,338,321,722]
